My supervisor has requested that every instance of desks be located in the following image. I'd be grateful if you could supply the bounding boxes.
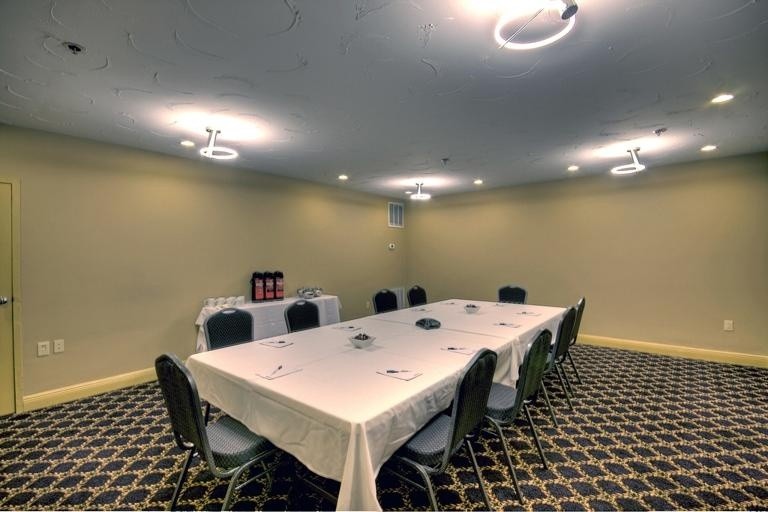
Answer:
[187,296,568,511]
[192,289,342,352]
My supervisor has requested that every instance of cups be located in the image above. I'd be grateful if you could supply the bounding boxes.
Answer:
[236,295,245,307]
[226,296,237,306]
[203,296,216,310]
[215,296,225,308]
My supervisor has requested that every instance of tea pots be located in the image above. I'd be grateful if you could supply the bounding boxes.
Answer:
[295,285,323,299]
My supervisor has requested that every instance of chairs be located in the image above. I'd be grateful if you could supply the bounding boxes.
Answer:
[498,283,528,303]
[531,297,586,431]
[155,354,275,512]
[480,330,561,506]
[406,284,427,307]
[284,297,320,333]
[373,288,398,314]
[201,308,253,353]
[391,348,498,511]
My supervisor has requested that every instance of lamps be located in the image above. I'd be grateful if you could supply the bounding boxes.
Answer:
[610,145,647,177]
[407,182,434,206]
[199,125,241,164]
[485,0,580,56]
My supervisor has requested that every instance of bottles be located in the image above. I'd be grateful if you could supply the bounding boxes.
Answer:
[263,272,275,303]
[251,272,265,303]
[274,271,285,302]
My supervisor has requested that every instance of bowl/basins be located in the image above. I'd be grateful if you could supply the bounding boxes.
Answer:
[347,333,377,349]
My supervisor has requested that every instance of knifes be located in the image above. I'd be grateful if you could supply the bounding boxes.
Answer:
[442,345,468,352]
[385,367,413,375]
[270,363,288,376]
[339,325,356,330]
[499,322,515,326]
[464,305,481,314]
[267,339,285,344]
